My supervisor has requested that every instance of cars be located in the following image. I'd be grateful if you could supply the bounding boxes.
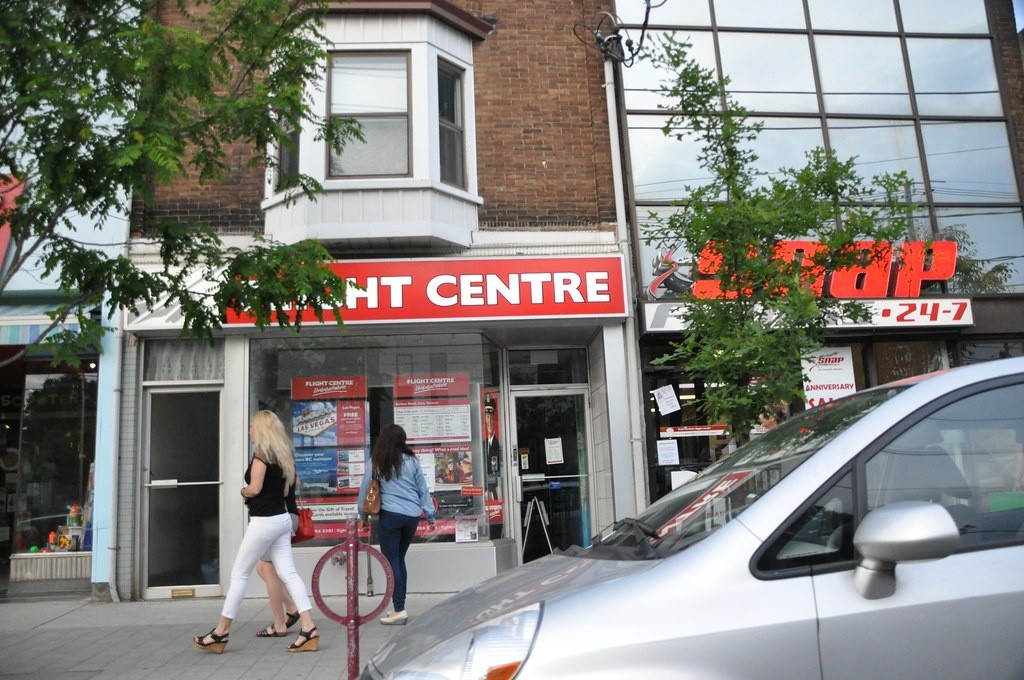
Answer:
[426,481,580,542]
[354,353,1023,679]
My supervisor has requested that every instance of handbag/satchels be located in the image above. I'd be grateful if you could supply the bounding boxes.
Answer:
[363,461,381,515]
[291,508,315,543]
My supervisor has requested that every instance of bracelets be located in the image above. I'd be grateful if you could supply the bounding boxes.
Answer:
[241,487,247,498]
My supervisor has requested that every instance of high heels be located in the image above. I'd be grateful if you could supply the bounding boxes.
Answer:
[287,626,320,652]
[193,628,229,654]
[380,609,408,625]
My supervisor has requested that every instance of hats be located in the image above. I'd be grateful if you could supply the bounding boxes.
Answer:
[483,392,496,412]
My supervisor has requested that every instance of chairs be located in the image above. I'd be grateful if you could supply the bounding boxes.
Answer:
[923,445,976,521]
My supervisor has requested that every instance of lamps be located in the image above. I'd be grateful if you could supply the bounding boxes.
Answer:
[574,11,634,67]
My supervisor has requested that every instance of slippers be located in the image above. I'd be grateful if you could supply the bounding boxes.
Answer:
[286,610,300,629]
[256,624,287,637]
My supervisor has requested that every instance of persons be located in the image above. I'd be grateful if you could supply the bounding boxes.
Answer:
[192,410,320,654]
[436,458,459,483]
[759,398,790,431]
[357,424,435,625]
[482,391,500,500]
